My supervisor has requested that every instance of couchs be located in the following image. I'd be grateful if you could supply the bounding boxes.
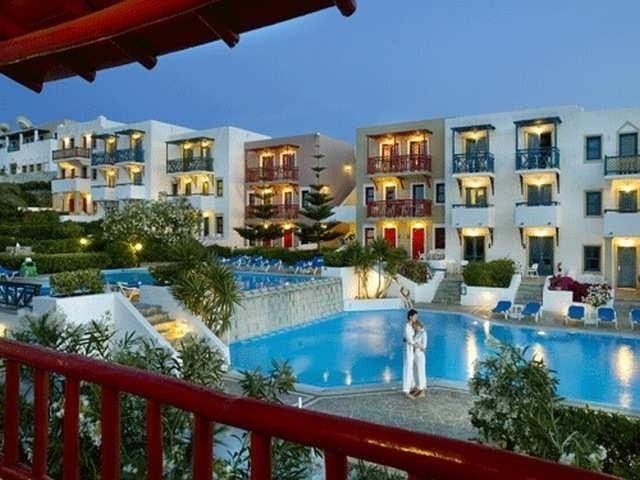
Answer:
[544,274,615,316]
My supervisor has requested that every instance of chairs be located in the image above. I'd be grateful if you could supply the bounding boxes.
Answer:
[219,254,324,276]
[528,263,541,280]
[486,300,639,330]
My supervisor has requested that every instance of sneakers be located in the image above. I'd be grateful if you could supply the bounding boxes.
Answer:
[406,390,426,400]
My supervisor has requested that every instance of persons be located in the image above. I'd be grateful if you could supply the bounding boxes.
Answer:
[402,307,424,401]
[411,319,428,398]
